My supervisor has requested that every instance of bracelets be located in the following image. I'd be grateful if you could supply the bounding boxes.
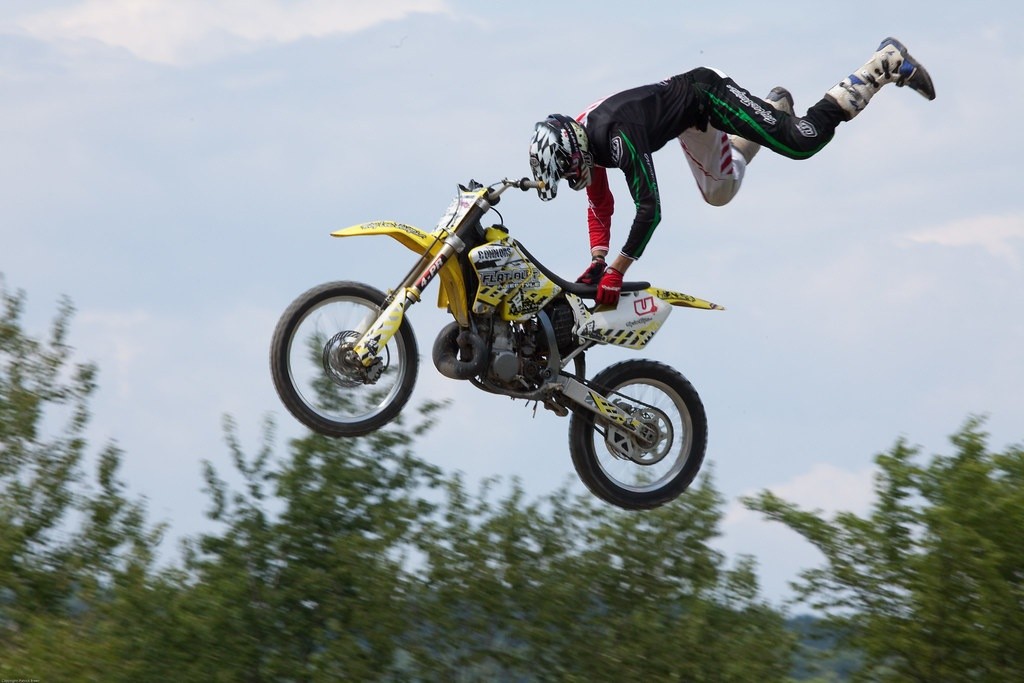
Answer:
[591,254,605,261]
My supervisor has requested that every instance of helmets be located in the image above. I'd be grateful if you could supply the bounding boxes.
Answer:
[529,113,594,201]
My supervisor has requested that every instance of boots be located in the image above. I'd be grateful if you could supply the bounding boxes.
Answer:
[824,36,935,122]
[730,87,795,165]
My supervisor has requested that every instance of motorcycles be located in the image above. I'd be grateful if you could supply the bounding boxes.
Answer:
[270,176,706,511]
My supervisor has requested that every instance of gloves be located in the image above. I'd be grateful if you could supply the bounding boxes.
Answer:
[595,266,624,306]
[575,255,608,285]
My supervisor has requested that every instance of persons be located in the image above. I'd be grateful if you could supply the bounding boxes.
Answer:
[529,38,936,307]
